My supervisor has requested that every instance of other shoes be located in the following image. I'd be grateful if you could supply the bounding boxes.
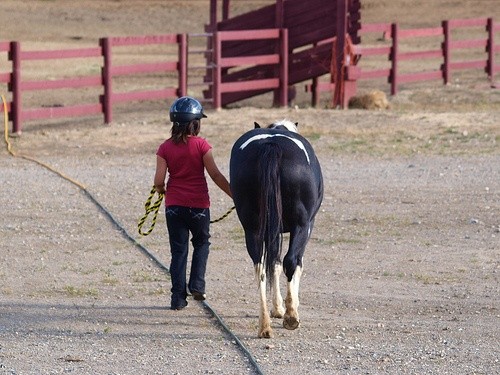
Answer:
[171,302,188,311]
[191,291,206,301]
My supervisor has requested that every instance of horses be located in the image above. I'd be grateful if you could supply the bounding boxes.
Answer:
[229,120,324,338]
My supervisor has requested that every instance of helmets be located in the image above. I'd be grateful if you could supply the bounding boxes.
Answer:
[170,97,208,122]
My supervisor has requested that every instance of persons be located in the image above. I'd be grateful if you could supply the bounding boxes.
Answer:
[154,96,233,310]
